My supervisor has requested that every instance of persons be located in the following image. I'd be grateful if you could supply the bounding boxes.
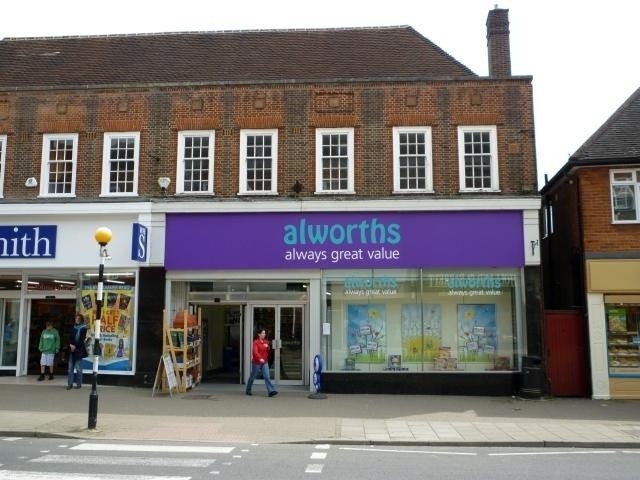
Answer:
[37,321,61,381]
[113,339,128,358]
[246,328,279,398]
[65,313,89,390]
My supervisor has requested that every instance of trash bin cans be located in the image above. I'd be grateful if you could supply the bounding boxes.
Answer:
[521,355,545,399]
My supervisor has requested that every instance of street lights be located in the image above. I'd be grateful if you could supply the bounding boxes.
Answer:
[88,226,112,429]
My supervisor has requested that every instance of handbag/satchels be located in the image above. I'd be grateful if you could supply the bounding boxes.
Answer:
[72,342,89,360]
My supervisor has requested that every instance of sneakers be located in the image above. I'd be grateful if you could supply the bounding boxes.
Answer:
[38,373,81,390]
[246,391,279,397]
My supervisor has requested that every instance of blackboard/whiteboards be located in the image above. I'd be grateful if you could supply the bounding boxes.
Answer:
[154,355,165,387]
[163,354,179,389]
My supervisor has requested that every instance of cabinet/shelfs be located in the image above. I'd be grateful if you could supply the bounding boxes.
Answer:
[608,331,640,367]
[161,308,202,393]
